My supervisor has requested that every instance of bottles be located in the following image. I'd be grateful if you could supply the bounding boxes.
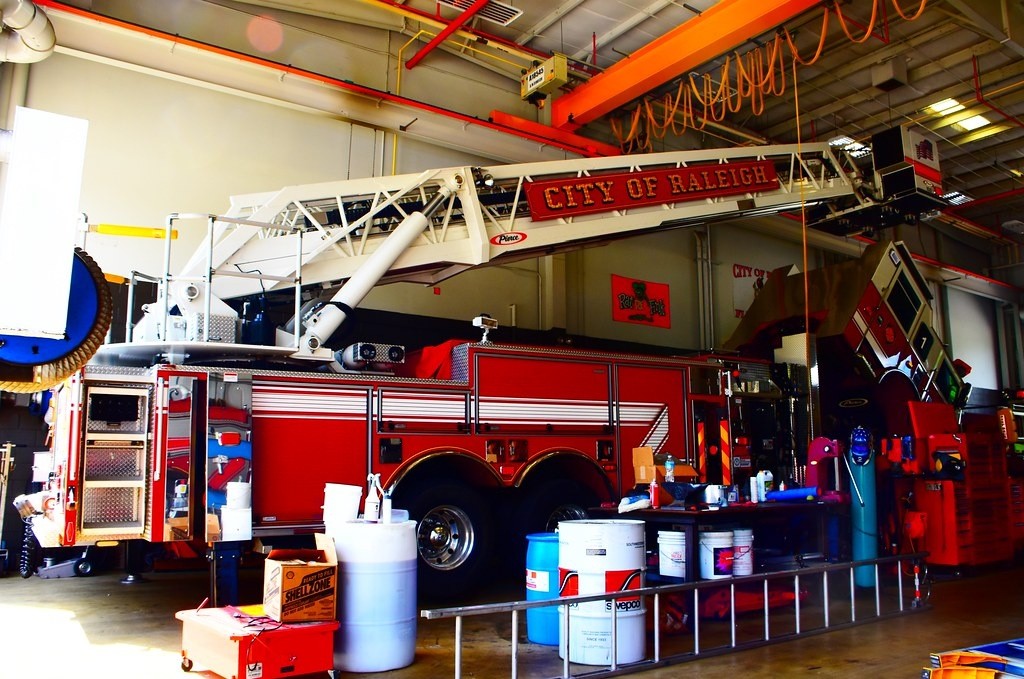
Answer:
[763,470,773,494]
[665,455,674,482]
[756,470,765,502]
[649,478,658,510]
[383,490,392,523]
[750,477,758,503]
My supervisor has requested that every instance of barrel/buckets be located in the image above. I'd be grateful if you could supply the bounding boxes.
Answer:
[558,519,646,665]
[657,528,753,579]
[525,532,559,645]
[324,521,417,673]
[322,483,362,519]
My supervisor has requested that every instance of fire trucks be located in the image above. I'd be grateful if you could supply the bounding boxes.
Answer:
[13,129,1024,609]
